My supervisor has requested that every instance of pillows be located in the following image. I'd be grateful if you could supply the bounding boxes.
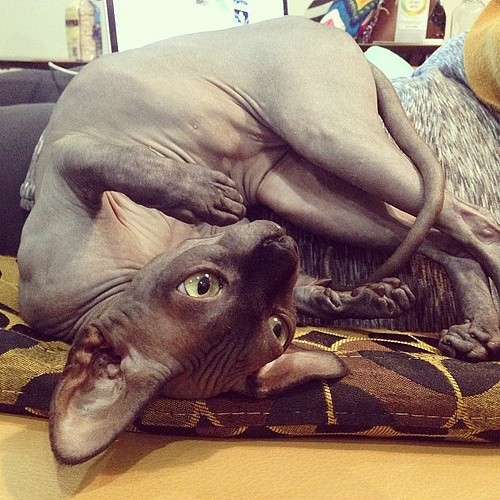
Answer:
[303,0,391,54]
[0,253,499,446]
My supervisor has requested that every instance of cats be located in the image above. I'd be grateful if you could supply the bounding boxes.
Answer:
[15,16,499,466]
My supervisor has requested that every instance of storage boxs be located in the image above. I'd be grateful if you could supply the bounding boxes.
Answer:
[373,1,430,45]
